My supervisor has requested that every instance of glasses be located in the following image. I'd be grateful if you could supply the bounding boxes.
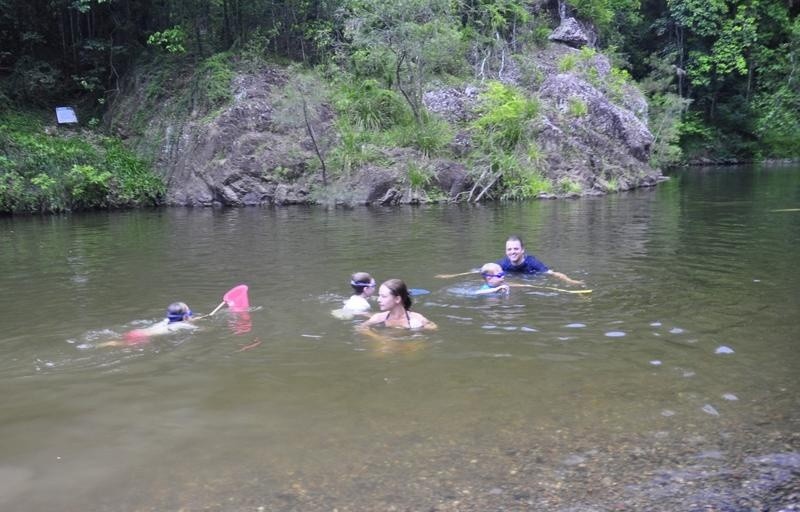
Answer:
[188,310,192,315]
[484,272,504,278]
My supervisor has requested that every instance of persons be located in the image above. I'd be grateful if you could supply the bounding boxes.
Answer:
[341,271,377,317]
[95,301,212,348]
[358,278,438,344]
[475,262,509,298]
[435,234,585,289]
